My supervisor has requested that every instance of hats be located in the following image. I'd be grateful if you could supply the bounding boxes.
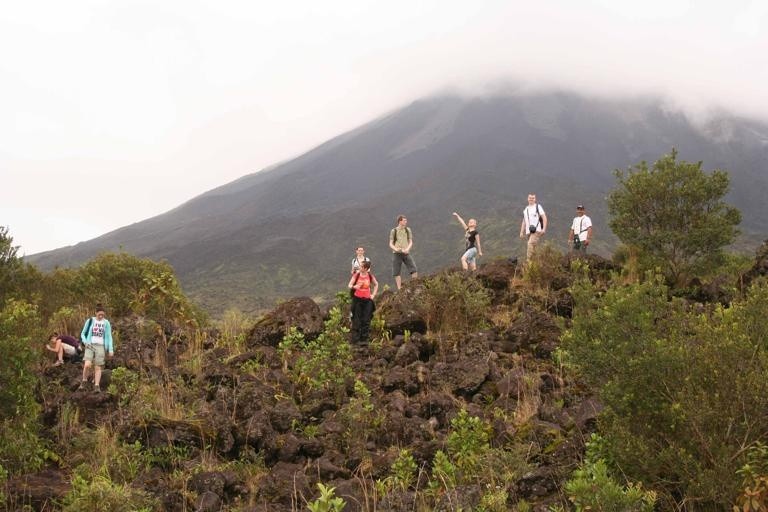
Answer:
[577,204,584,209]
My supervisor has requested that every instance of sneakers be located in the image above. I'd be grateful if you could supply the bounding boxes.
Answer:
[54,360,64,366]
[95,385,101,393]
[79,381,87,390]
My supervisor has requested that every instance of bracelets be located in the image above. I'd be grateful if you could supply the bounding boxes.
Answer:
[351,286,354,290]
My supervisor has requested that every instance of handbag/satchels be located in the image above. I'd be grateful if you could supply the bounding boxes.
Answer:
[573,234,580,249]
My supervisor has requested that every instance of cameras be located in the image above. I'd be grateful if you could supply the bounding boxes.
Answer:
[529,227,536,233]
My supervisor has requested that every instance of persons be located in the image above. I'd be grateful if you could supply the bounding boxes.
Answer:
[77,303,114,393]
[44,332,82,367]
[566,204,592,253]
[350,246,370,274]
[388,214,417,290]
[348,261,378,345]
[518,193,547,263]
[451,211,482,271]
